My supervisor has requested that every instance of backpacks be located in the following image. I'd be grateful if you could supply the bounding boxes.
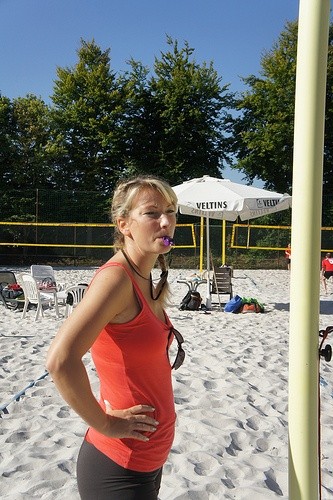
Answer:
[224,295,243,312]
[0,282,24,299]
[38,277,59,292]
[178,290,203,311]
[186,273,201,281]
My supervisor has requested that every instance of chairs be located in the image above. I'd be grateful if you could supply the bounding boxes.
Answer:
[0,264,88,322]
[209,268,233,301]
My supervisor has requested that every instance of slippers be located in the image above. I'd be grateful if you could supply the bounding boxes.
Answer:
[199,310,211,314]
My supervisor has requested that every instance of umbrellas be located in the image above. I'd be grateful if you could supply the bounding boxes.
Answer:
[171,175,292,309]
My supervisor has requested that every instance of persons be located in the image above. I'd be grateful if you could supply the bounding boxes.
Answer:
[46,179,176,500]
[284,244,291,270]
[322,252,333,296]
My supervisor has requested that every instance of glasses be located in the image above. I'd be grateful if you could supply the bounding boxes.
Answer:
[166,326,186,371]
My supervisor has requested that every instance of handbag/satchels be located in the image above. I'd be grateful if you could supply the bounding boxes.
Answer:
[67,283,89,306]
[240,302,261,314]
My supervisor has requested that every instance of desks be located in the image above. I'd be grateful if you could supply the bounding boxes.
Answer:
[177,280,207,290]
